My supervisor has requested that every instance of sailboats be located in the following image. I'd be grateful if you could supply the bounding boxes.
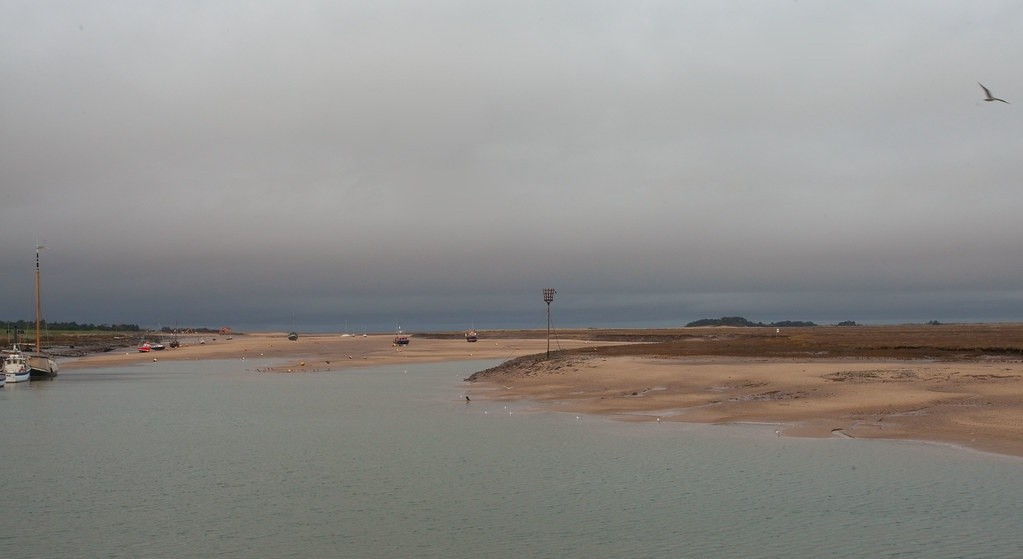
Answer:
[467,320,478,342]
[138,329,151,352]
[288,312,297,341]
[152,322,165,351]
[392,325,409,346]
[26,239,59,378]
[169,320,180,348]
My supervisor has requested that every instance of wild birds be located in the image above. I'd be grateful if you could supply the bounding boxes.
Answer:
[979,82,1012,105]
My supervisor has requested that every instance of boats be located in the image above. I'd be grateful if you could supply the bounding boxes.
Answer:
[0,368,6,386]
[5,345,33,383]
